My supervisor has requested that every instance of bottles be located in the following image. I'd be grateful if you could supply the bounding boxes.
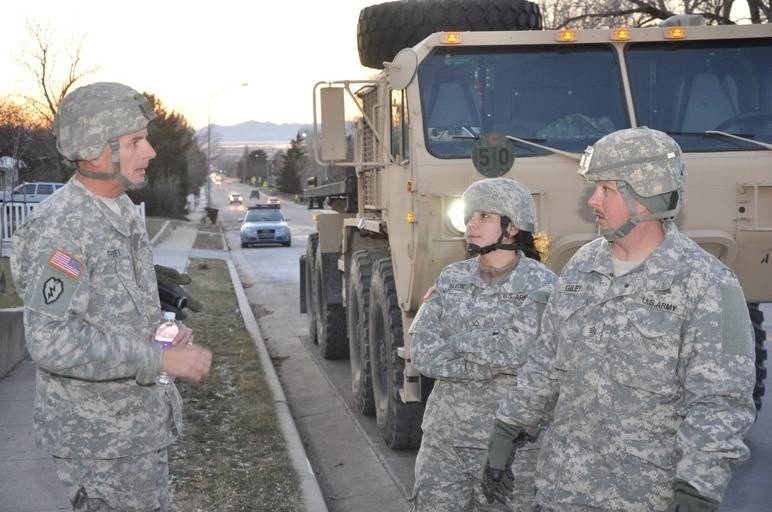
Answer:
[153,310,180,386]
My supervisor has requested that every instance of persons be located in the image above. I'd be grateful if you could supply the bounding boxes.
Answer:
[477,125,757,512]
[405,178,561,512]
[10,82,212,512]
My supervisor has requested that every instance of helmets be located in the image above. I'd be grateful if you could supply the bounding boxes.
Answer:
[577,123,684,240]
[461,175,536,254]
[51,81,161,190]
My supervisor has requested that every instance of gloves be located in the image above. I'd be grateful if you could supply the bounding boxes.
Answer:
[154,263,204,323]
[663,478,725,512]
[480,416,523,506]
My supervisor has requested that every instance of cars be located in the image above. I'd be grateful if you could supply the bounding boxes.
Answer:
[250,190,260,201]
[266,198,281,208]
[0,180,67,210]
[238,204,294,247]
[210,170,243,205]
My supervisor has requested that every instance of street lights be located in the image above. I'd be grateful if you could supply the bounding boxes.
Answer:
[207,82,249,196]
[254,154,271,190]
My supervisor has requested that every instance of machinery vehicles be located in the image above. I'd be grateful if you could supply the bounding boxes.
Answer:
[299,0,771,452]
